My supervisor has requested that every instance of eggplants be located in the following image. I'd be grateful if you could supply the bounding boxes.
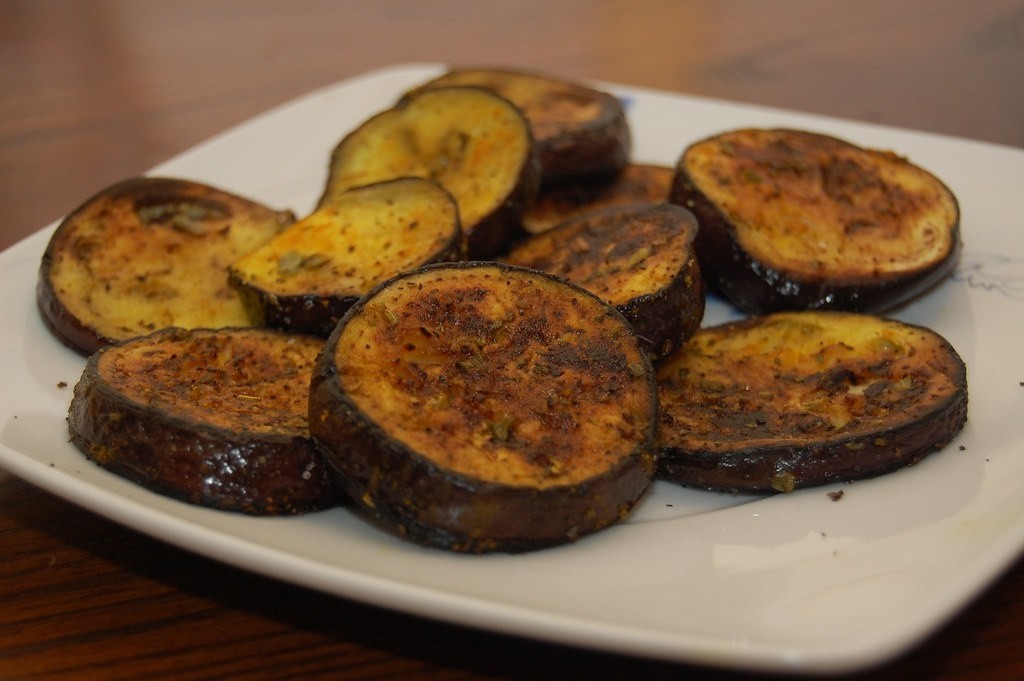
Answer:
[39,68,970,559]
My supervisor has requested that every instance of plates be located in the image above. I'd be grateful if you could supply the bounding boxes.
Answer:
[1,59,1023,666]
